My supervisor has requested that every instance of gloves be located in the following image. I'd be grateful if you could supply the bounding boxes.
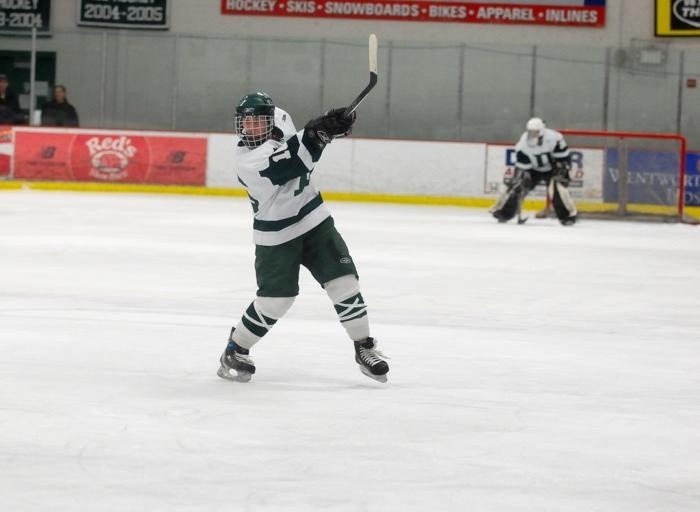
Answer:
[313,107,356,144]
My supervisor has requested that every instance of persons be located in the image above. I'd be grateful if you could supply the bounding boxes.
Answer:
[39,84,80,128]
[492,118,580,222]
[219,90,389,376]
[0,74,22,126]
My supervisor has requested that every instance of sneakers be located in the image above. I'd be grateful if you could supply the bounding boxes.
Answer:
[354,338,389,375]
[220,340,255,373]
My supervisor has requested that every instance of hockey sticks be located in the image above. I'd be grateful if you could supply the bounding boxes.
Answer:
[518,185,528,225]
[342,34,378,115]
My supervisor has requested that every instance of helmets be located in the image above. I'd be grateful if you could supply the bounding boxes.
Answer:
[526,117,546,137]
[234,92,275,149]
